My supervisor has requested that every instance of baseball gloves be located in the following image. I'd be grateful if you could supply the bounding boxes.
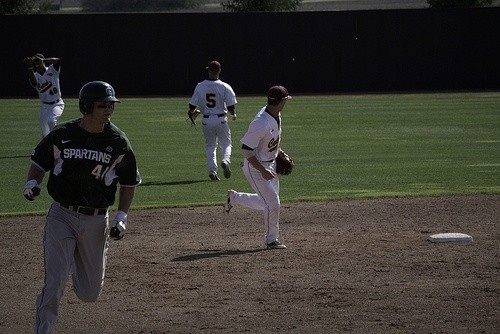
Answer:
[275,153,294,175]
[188,106,200,124]
[21,56,43,73]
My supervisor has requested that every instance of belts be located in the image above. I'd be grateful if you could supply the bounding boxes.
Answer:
[203,113,225,118]
[60,202,107,215]
[42,99,59,104]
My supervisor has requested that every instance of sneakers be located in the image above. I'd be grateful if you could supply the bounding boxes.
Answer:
[209,172,220,180]
[221,161,231,179]
[267,239,287,249]
[224,190,236,214]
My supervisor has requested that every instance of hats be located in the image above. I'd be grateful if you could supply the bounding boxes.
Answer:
[268,86,292,100]
[206,61,221,71]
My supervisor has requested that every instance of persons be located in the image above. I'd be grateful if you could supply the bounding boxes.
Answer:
[224,86,294,250]
[188,61,237,181]
[23,53,64,140]
[24,81,143,334]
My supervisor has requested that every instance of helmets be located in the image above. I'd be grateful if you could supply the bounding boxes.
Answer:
[79,81,121,115]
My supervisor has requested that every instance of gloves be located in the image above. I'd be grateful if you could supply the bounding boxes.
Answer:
[110,211,127,241]
[23,179,40,201]
